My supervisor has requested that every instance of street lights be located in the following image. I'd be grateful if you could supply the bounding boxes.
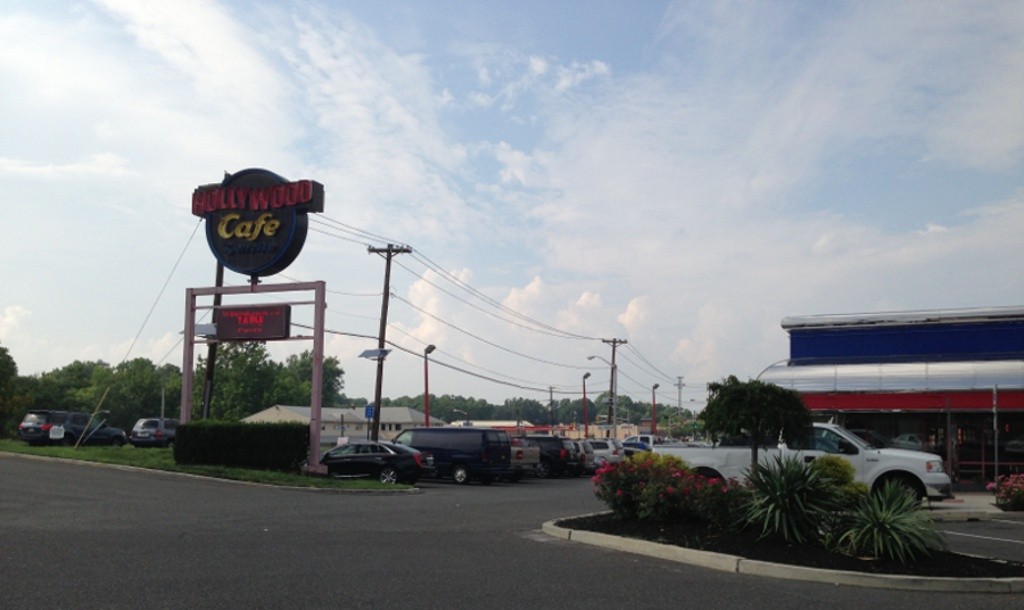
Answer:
[588,355,617,438]
[652,384,660,436]
[424,345,436,429]
[582,373,591,438]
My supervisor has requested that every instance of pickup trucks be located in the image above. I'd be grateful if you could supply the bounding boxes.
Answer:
[657,421,950,507]
[854,430,922,451]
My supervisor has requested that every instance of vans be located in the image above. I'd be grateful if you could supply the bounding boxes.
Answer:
[391,427,510,485]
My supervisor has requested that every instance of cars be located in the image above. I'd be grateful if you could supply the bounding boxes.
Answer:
[20,409,129,448]
[321,441,428,485]
[892,433,927,450]
[510,435,685,480]
[129,417,178,448]
